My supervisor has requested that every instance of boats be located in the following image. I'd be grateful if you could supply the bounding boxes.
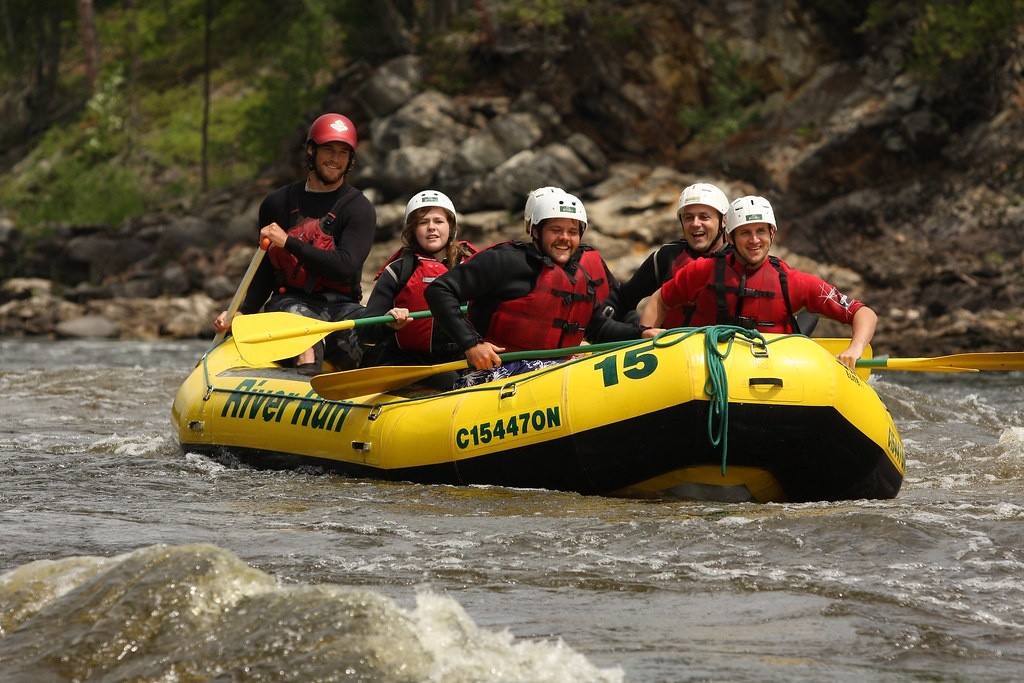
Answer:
[171,328,904,503]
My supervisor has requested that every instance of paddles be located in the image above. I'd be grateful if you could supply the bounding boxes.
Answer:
[194,236,271,369]
[229,304,469,368]
[835,350,1024,375]
[808,335,876,384]
[309,337,646,402]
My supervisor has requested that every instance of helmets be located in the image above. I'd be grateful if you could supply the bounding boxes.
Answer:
[676,182,730,219]
[530,193,588,236]
[306,112,358,152]
[524,186,567,223]
[725,195,777,235]
[404,189,458,225]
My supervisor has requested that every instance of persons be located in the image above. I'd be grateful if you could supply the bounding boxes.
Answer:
[618,180,730,321]
[213,113,377,370]
[424,186,667,390]
[639,194,878,370]
[356,189,480,390]
